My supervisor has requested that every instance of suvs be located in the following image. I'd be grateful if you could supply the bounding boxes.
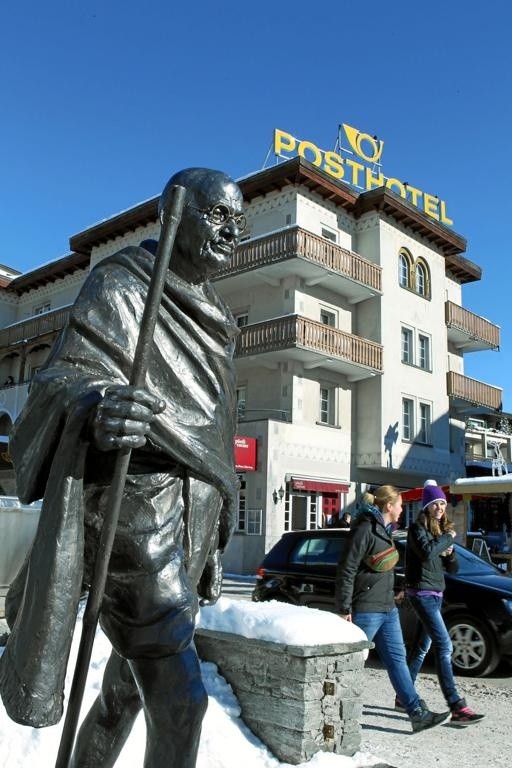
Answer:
[253,529,511,676]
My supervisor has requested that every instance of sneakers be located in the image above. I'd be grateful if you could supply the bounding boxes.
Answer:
[449,699,486,726]
[411,700,450,732]
[394,698,407,712]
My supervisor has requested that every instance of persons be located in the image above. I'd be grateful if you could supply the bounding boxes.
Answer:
[395,479,486,726]
[335,485,452,734]
[334,513,351,528]
[1,165,245,768]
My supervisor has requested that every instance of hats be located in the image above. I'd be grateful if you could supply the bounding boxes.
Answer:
[421,479,448,511]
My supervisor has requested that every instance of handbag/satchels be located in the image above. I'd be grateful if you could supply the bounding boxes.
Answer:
[363,541,400,572]
